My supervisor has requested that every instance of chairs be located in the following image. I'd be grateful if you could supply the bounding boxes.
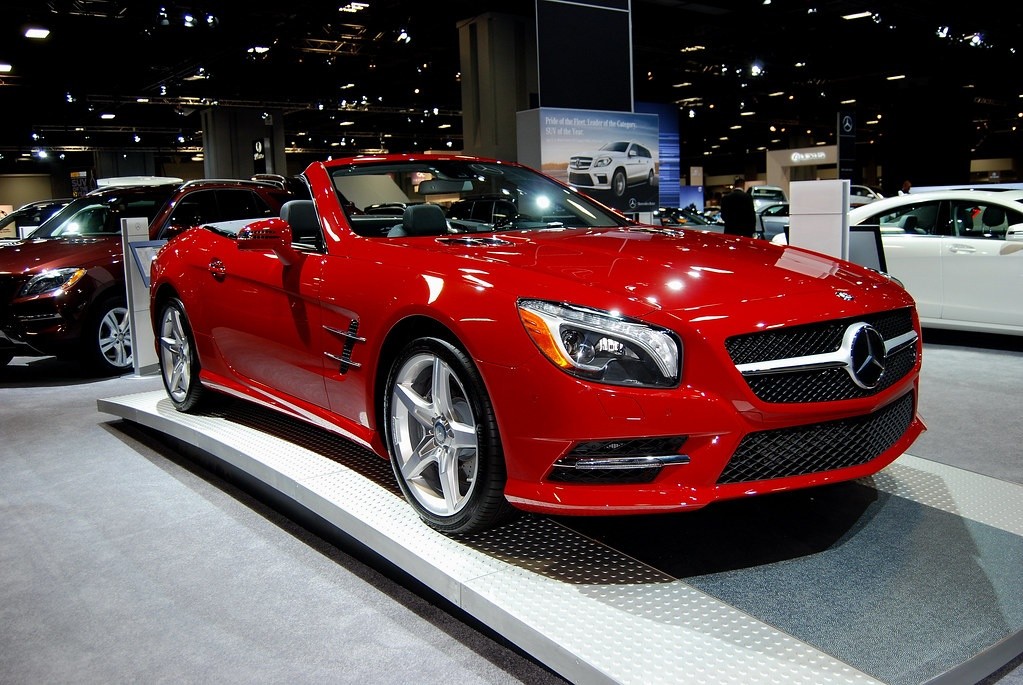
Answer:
[388,204,463,239]
[899,215,919,228]
[980,207,1005,237]
[282,199,324,248]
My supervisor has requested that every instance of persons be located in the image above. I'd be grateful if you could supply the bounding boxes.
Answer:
[721,177,756,237]
[897,181,911,195]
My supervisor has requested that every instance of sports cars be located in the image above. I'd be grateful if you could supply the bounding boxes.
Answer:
[147,152,928,538]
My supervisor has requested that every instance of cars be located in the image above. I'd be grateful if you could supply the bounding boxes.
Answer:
[771,189,1023,337]
[653,184,914,236]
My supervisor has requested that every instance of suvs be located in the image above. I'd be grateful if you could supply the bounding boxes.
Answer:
[0,176,298,377]
[0,198,161,242]
[565,141,657,198]
